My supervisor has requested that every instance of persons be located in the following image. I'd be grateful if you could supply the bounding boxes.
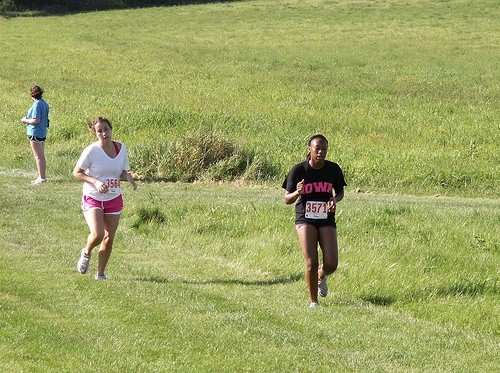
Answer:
[282,134,348,310]
[73,116,137,281]
[20,85,49,185]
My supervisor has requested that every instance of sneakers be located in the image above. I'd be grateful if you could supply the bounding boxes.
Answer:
[97,272,106,280]
[311,300,318,307]
[318,278,328,297]
[32,177,47,184]
[78,248,91,274]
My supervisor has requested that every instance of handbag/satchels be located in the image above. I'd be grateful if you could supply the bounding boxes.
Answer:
[47,119,50,127]
